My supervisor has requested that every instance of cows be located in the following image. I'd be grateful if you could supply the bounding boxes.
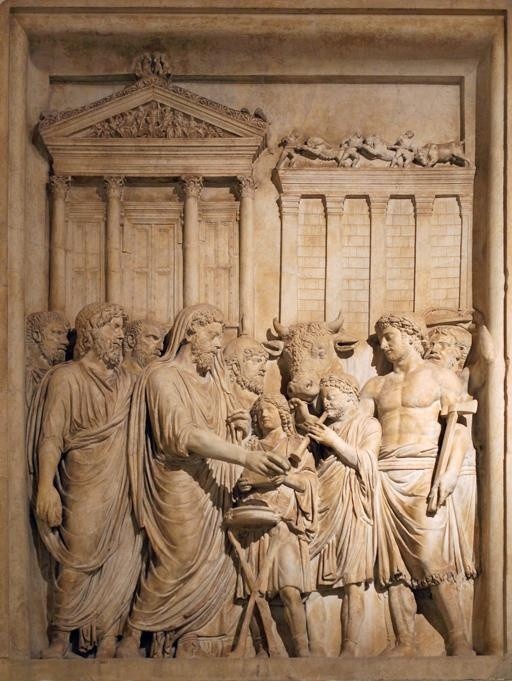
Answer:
[262,307,359,402]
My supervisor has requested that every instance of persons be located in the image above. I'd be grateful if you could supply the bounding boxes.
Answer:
[27,303,495,658]
